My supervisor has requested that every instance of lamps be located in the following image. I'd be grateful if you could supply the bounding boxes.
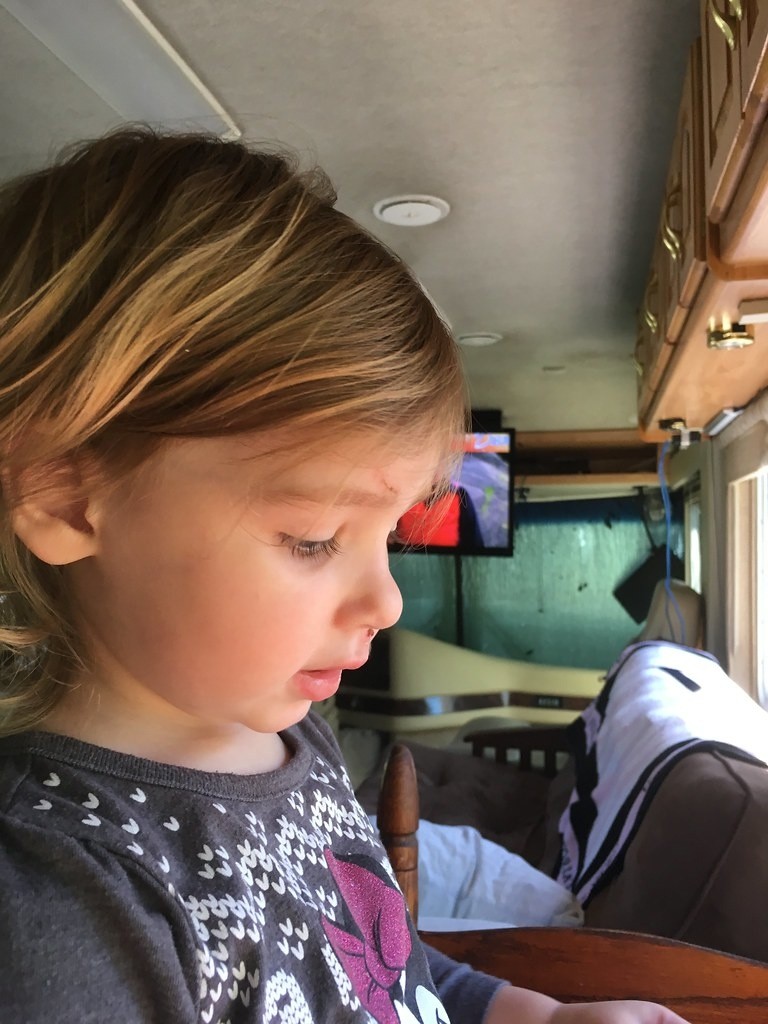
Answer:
[0,0,241,146]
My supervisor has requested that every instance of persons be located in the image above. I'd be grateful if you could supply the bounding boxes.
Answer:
[0,125,688,1022]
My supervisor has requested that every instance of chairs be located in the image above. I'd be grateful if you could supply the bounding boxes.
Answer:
[374,744,768,1024]
[350,637,768,964]
[441,578,705,775]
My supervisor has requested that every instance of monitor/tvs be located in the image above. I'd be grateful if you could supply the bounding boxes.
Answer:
[384,427,517,559]
